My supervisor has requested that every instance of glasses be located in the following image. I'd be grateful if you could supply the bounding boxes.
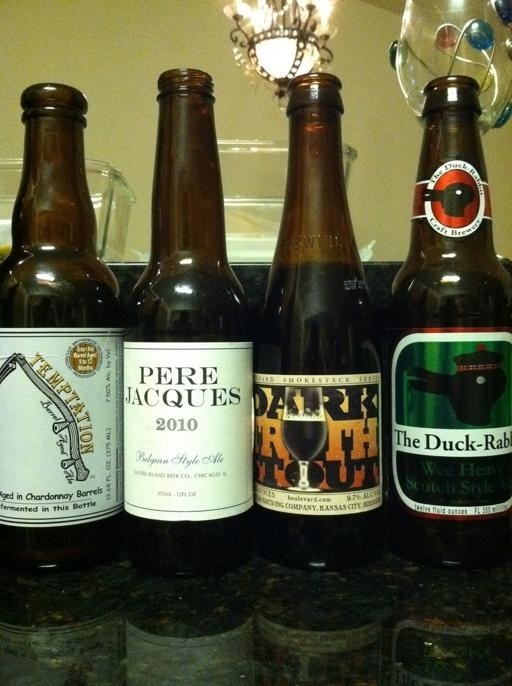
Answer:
[222,1,339,98]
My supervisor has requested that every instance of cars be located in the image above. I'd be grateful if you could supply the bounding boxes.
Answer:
[395,0,511,138]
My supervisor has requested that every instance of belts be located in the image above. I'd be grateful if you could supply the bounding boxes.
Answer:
[280,387,328,492]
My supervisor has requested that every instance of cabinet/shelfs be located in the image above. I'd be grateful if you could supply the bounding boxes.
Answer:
[384,75,512,566]
[253,72,387,567]
[124,68,253,567]
[1,83,124,566]
